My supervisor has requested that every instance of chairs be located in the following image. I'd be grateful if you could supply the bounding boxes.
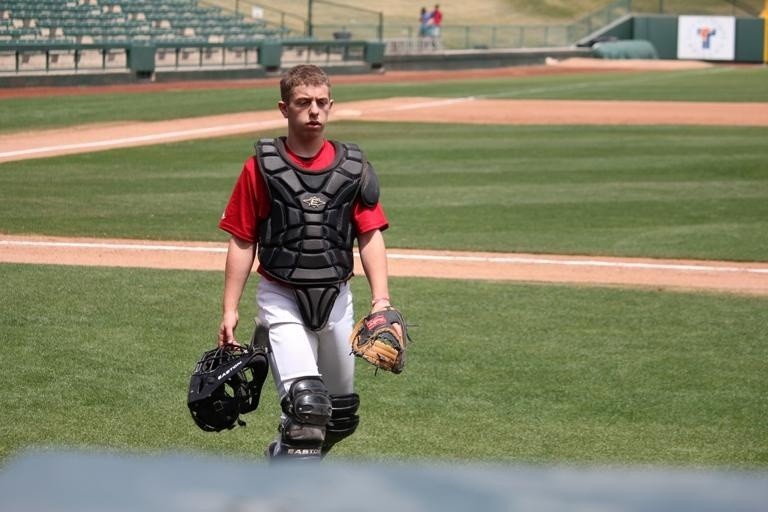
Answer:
[0,2,307,64]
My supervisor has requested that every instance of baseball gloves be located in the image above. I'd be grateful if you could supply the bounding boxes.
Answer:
[348,307,409,375]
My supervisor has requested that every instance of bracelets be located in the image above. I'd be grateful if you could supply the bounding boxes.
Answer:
[372,294,390,307]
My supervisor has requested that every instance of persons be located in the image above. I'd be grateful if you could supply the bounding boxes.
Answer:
[216,64,393,460]
[426,4,442,48]
[418,7,432,48]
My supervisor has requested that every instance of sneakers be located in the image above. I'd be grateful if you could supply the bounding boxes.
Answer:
[263,441,294,493]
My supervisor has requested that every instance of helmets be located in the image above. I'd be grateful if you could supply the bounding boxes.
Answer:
[186,344,270,433]
